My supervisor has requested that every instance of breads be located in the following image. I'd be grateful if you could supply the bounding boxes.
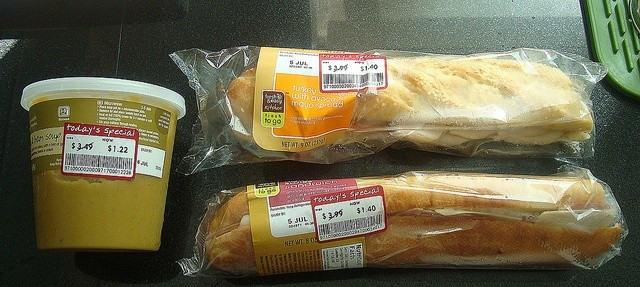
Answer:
[206,173,623,275]
[226,57,593,150]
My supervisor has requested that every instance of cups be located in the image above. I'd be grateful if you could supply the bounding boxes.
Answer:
[20,76,187,251]
